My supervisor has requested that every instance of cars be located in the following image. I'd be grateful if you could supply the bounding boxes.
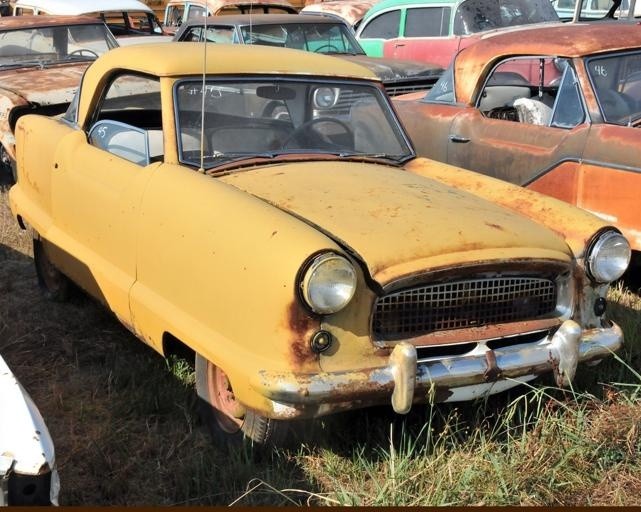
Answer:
[0,14,187,187]
[0,0,175,56]
[8,41,632,465]
[350,17,641,293]
[162,0,641,136]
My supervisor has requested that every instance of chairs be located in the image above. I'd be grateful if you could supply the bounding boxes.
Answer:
[102,126,301,166]
